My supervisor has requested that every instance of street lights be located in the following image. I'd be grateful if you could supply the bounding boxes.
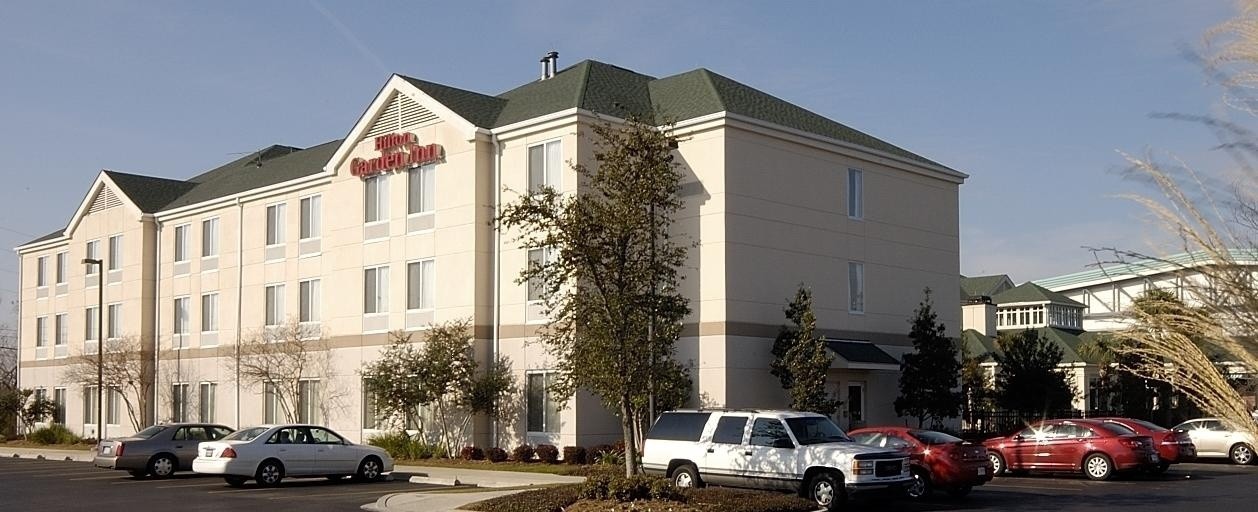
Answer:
[80,257,102,446]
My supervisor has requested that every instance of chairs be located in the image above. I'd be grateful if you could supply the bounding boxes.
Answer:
[281,431,291,443]
[294,434,304,443]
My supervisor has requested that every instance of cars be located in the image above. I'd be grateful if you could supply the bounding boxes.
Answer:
[191,423,396,488]
[845,427,994,502]
[639,406,917,510]
[981,418,1162,481]
[1171,411,1257,466]
[1097,416,1199,472]
[93,422,236,479]
